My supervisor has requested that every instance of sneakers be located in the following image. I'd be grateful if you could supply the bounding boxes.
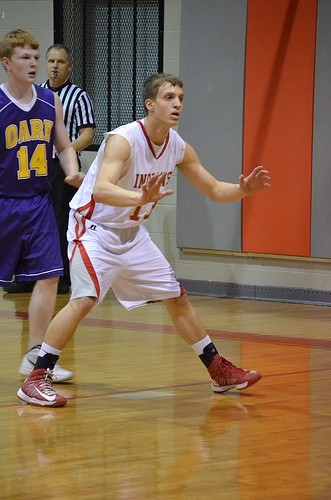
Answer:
[16,367,68,407]
[207,353,262,393]
[18,348,75,382]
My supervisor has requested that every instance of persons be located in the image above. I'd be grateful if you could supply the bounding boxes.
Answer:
[0,28,87,382]
[2,44,96,294]
[15,72,272,407]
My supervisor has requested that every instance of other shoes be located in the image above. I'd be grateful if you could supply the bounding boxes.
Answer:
[57,283,69,295]
[3,280,35,294]
